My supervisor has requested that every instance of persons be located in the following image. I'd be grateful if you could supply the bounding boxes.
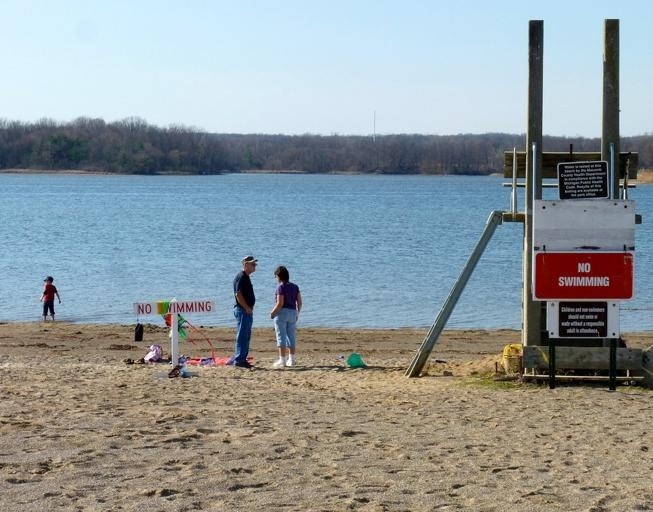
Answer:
[269,266,301,368]
[39,275,61,321]
[231,255,258,367]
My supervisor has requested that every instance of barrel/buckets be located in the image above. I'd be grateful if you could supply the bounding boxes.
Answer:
[134,323,143,341]
[503,354,521,372]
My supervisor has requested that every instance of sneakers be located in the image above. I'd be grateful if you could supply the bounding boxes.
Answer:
[273,360,286,367]
[234,360,254,368]
[286,359,297,367]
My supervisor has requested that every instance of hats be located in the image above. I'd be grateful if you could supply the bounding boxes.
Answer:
[241,255,258,265]
